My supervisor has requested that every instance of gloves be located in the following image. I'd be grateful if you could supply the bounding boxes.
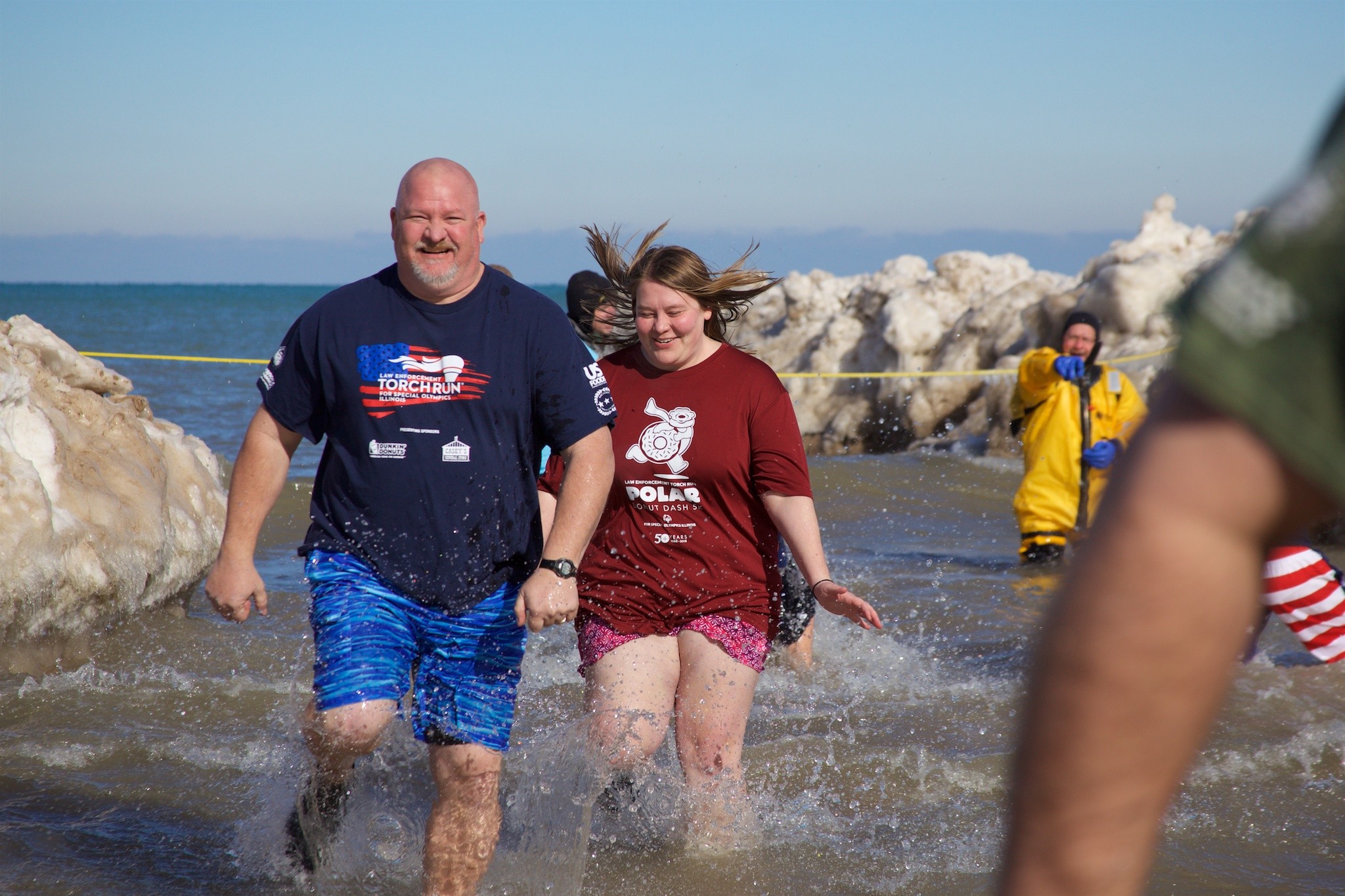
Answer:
[1082,441,1118,470]
[1055,355,1084,381]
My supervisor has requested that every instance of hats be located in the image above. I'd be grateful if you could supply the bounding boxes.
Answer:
[1061,310,1102,342]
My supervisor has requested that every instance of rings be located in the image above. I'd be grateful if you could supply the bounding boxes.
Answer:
[555,617,567,625]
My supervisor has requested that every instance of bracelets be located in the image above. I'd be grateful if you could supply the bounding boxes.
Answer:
[812,579,835,601]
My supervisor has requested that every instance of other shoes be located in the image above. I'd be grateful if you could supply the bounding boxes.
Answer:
[288,775,348,873]
[596,773,636,812]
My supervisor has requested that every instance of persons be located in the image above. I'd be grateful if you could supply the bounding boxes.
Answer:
[995,94,1345,896]
[1263,541,1345,666]
[539,270,620,477]
[1008,309,1152,567]
[204,156,616,896]
[532,221,885,809]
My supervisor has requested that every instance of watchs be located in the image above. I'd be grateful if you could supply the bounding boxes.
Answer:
[538,557,579,578]
